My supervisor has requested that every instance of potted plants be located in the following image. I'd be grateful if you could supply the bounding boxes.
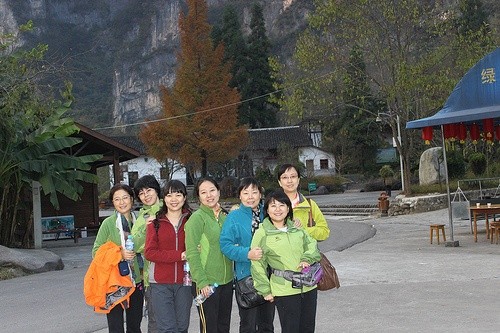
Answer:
[379,165,394,184]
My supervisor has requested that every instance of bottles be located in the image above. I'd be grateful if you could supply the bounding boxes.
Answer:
[126,234,133,264]
[118,259,129,276]
[192,283,218,306]
[182,260,192,287]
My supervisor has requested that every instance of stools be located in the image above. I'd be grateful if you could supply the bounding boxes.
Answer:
[430,224,446,244]
[489,221,500,244]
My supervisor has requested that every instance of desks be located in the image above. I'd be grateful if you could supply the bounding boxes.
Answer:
[467,204,500,242]
[43,228,80,244]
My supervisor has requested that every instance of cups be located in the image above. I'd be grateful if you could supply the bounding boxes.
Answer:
[487,203,491,207]
[476,203,480,208]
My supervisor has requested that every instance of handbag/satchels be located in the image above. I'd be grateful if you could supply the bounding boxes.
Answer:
[313,253,340,291]
[272,262,323,289]
[234,272,269,310]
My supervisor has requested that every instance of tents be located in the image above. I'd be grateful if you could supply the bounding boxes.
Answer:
[406,46,499,241]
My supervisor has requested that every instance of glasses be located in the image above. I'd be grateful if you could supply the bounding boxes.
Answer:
[111,195,130,203]
[280,173,298,180]
[138,188,154,198]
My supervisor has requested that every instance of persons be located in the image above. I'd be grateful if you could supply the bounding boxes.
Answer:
[218,179,275,333]
[275,165,330,241]
[146,180,193,333]
[251,193,321,332]
[184,175,235,333]
[91,185,144,333]
[133,174,162,310]
